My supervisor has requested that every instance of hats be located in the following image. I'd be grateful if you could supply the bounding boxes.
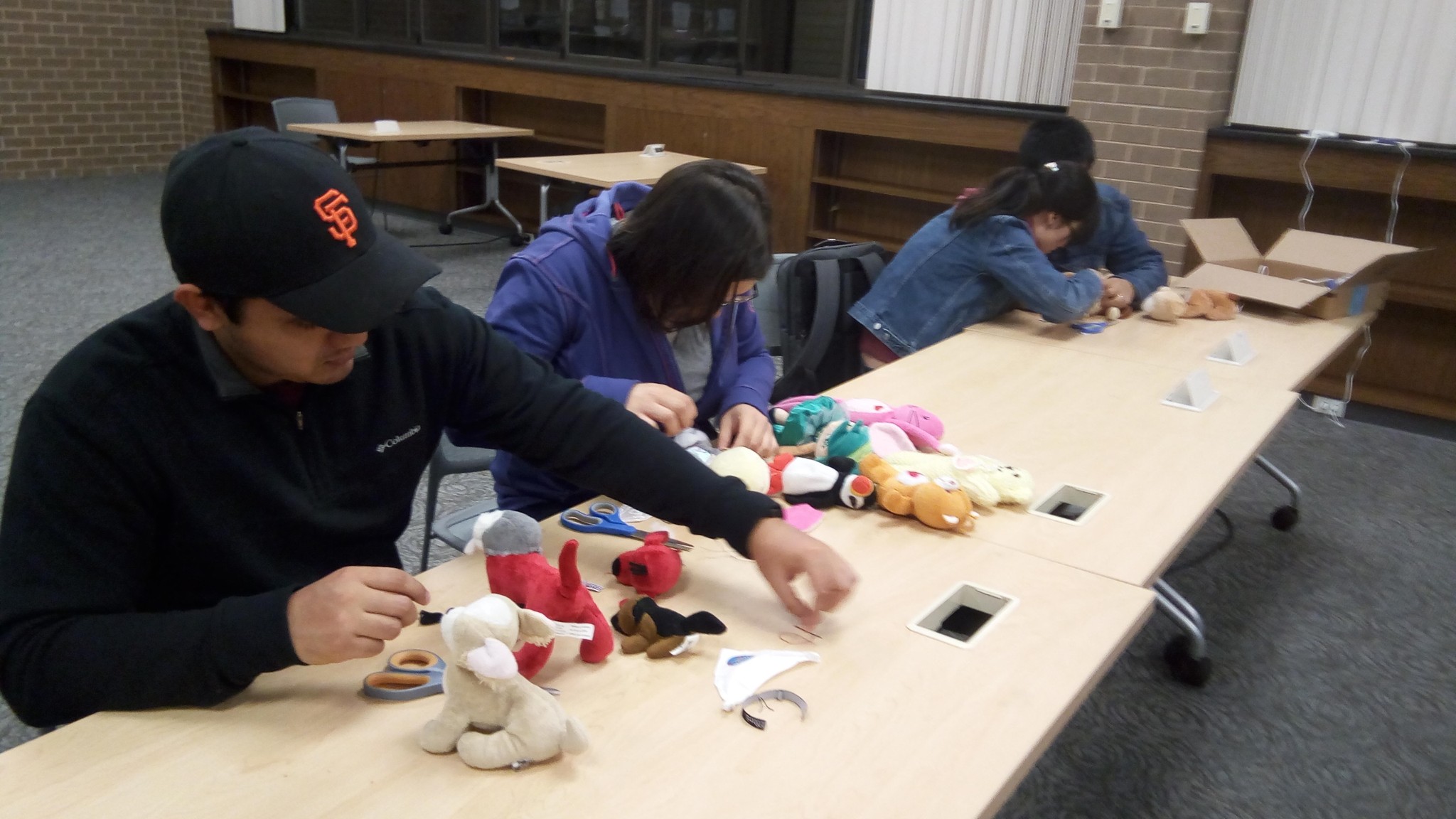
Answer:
[158,127,443,335]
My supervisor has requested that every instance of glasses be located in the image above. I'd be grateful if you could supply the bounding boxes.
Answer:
[721,283,761,306]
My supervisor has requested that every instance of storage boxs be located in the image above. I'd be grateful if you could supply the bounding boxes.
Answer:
[1180,218,1419,322]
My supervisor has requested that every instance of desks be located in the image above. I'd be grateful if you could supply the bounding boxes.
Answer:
[287,119,535,246]
[2,491,1159,819]
[495,148,768,227]
[965,265,1378,536]
[811,329,1303,688]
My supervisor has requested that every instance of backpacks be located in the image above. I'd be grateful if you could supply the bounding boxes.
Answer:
[768,237,890,406]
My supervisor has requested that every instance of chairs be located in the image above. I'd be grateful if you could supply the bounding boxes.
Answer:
[271,97,392,234]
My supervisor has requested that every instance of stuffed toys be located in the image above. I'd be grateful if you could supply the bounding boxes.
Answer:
[419,593,588,771]
[610,595,727,659]
[767,452,875,511]
[870,421,1030,505]
[1144,285,1238,321]
[773,398,867,465]
[684,445,821,530]
[858,455,979,527]
[610,530,681,597]
[770,395,955,456]
[464,509,614,681]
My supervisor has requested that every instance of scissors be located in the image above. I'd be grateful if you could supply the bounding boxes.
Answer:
[560,501,694,553]
[1071,321,1108,334]
[364,648,561,699]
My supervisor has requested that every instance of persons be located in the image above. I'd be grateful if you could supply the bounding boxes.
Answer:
[1001,117,1169,314]
[1,128,857,736]
[847,158,1109,377]
[486,158,776,522]
